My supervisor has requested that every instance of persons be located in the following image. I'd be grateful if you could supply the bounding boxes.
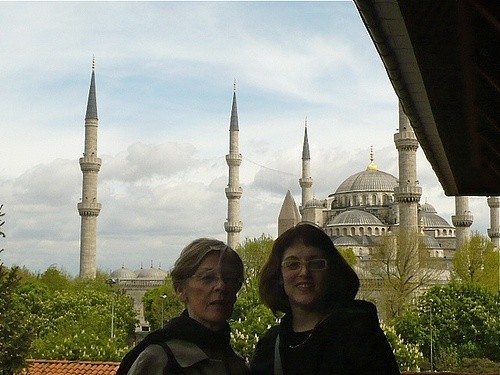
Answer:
[117,238,251,375]
[251,221,401,375]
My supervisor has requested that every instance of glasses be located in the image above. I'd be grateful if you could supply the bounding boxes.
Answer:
[191,271,244,290]
[280,258,330,272]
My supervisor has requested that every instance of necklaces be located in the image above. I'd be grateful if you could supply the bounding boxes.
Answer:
[288,319,326,349]
